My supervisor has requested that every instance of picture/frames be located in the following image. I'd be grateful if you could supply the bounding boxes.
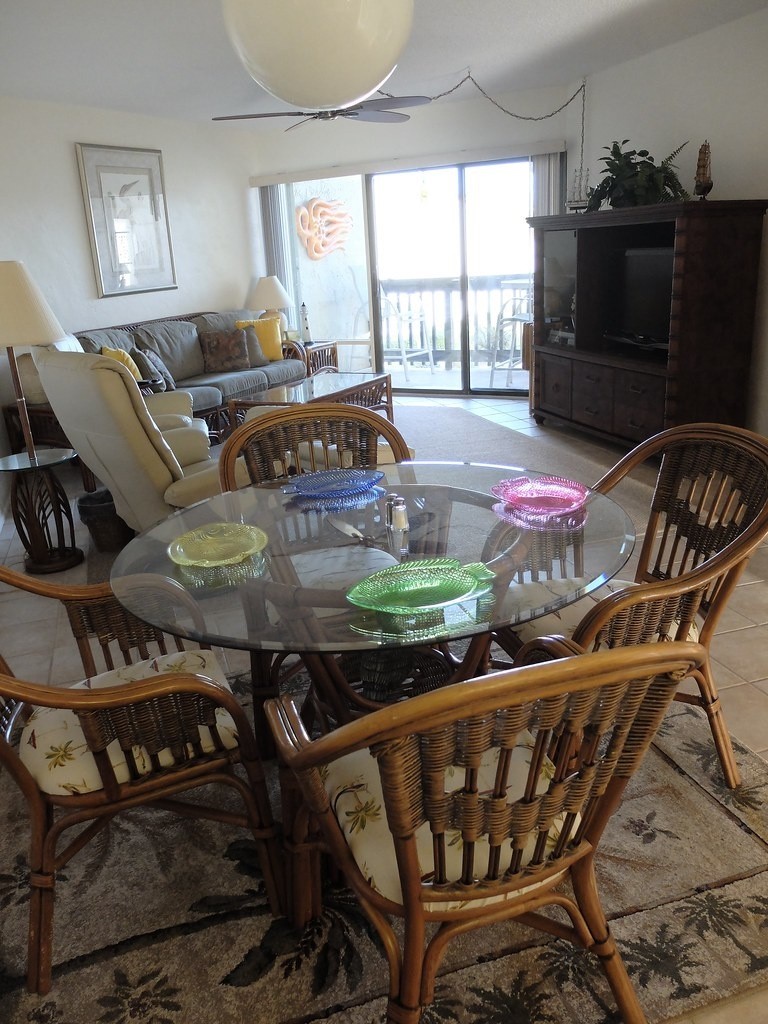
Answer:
[74,142,178,298]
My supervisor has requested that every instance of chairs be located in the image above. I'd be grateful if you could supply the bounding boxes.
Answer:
[1,404,768,1024]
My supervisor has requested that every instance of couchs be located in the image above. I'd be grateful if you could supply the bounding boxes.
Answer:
[73,310,308,443]
[31,338,430,542]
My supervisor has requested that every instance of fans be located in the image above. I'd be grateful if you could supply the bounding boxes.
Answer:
[213,96,432,133]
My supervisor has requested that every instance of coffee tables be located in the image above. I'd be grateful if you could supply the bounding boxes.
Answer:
[229,370,393,456]
[110,458,639,930]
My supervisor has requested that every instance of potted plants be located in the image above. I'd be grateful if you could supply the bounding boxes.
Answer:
[583,139,692,214]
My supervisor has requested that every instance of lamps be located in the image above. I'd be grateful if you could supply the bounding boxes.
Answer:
[220,0,416,110]
[250,276,296,341]
[0,261,71,459]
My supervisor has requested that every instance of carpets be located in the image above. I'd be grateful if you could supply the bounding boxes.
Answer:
[0,635,767,1024]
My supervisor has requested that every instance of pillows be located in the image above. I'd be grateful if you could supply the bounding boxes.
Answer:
[103,316,283,394]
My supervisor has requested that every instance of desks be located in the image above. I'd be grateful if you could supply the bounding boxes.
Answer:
[283,342,339,375]
[0,448,83,575]
[1,405,96,492]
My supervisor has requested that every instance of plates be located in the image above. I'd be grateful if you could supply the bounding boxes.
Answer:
[167,523,267,568]
[280,469,385,498]
[345,558,496,615]
[491,477,592,516]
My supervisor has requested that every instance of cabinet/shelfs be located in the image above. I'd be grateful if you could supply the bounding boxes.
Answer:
[526,199,768,462]
